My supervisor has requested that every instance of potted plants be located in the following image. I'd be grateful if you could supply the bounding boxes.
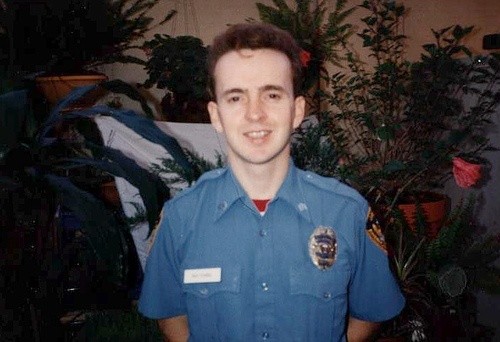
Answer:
[137,32,214,124]
[18,0,176,98]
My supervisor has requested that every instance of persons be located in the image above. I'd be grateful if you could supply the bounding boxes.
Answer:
[139,24,405,342]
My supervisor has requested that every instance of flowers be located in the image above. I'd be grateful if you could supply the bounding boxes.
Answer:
[305,1,500,195]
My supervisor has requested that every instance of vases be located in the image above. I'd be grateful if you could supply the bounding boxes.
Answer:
[368,185,450,242]
